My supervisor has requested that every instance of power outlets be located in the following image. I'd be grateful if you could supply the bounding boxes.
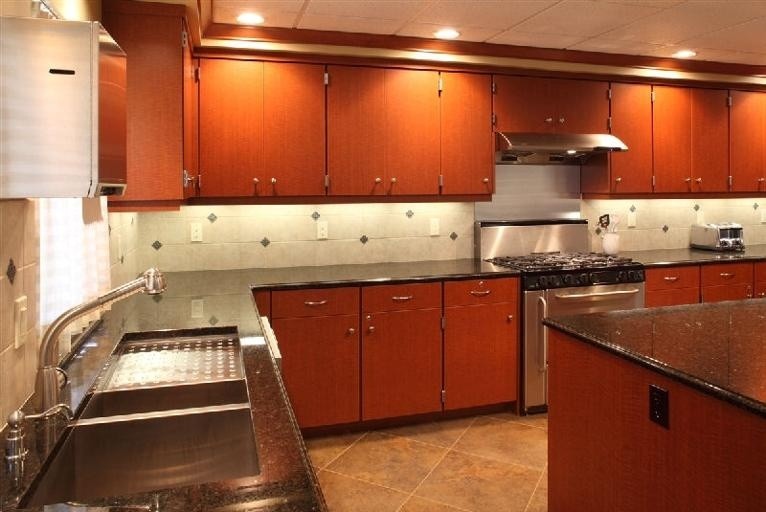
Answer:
[647,380,669,427]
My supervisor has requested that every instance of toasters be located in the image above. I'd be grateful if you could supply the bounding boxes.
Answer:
[690,222,746,251]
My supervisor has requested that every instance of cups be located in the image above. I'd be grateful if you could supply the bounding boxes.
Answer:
[601,233,620,255]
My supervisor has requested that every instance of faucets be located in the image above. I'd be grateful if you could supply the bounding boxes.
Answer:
[27,403,75,426]
[40,267,166,367]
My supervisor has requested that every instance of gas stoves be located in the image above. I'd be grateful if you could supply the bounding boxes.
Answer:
[483,250,646,291]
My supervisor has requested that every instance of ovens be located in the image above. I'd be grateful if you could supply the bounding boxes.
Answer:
[521,283,647,417]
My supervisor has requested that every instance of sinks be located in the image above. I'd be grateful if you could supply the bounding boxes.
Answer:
[0,408,265,510]
[73,377,254,423]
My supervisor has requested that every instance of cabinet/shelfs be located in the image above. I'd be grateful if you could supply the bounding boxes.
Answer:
[255,289,273,320]
[488,62,608,137]
[580,71,765,202]
[178,39,197,204]
[442,273,523,421]
[194,43,329,208]
[434,54,497,205]
[644,260,766,307]
[272,277,444,437]
[324,51,441,204]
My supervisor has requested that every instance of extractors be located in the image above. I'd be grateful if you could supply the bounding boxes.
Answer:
[493,130,630,167]
